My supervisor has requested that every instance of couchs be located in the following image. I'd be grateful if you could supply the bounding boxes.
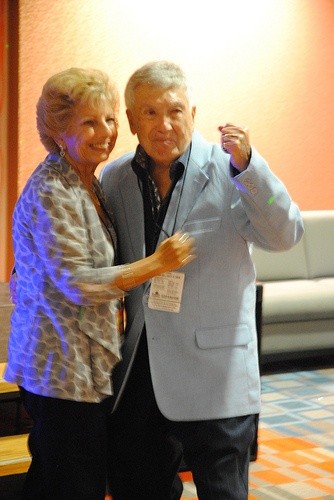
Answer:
[250,211,334,364]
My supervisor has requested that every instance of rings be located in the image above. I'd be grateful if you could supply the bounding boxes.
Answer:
[224,133,228,141]
[223,142,227,149]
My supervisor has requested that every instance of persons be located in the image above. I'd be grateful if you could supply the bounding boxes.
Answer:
[3,66,195,500]
[8,58,306,500]
